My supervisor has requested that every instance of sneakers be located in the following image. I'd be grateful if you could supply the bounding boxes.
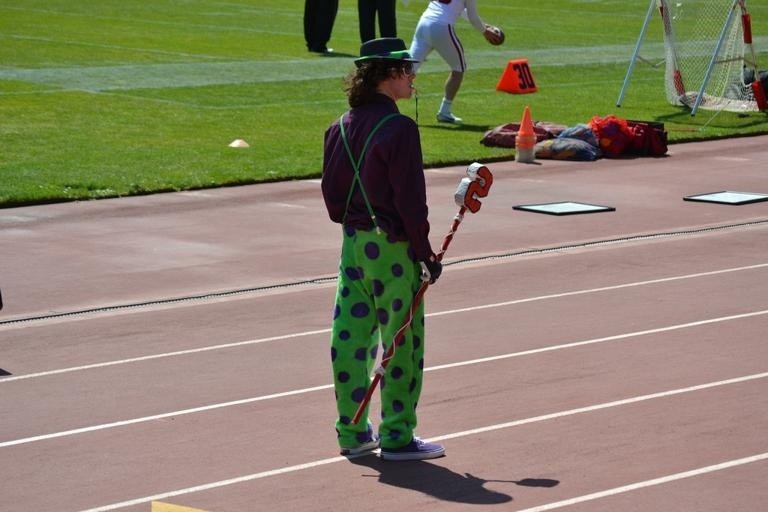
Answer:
[339,431,382,456]
[379,436,446,462]
[436,111,464,125]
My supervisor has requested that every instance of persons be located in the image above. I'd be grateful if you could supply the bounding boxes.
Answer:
[358,1,398,43]
[303,0,340,53]
[410,1,502,126]
[324,38,445,460]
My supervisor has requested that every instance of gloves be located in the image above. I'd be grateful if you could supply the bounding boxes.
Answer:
[418,251,443,286]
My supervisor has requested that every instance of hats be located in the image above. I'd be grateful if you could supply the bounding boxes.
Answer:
[353,35,421,65]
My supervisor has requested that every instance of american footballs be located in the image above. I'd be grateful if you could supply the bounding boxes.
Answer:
[483,26,504,45]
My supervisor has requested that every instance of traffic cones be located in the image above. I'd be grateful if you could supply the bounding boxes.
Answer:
[515,105,537,148]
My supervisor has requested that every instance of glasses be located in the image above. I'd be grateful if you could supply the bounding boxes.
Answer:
[390,62,414,77]
